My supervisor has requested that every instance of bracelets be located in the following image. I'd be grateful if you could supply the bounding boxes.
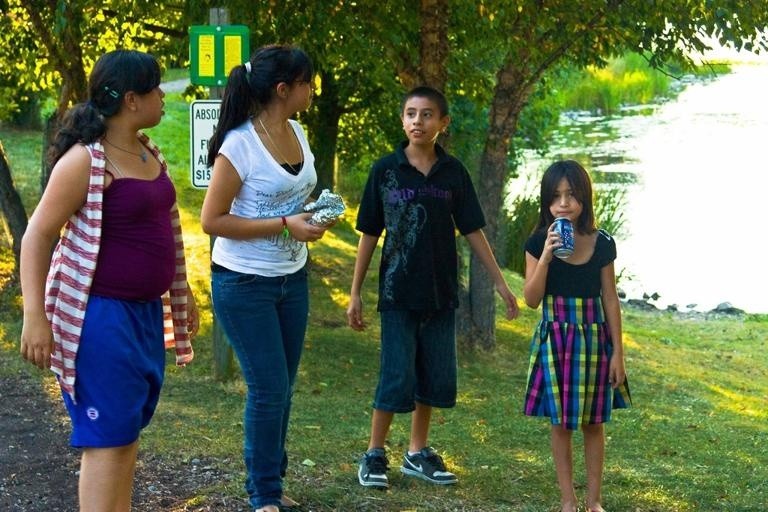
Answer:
[280,216,291,240]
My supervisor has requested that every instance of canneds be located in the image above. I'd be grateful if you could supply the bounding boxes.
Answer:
[554,217,575,258]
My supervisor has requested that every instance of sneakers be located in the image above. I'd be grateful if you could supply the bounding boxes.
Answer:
[358,447,390,488]
[400,446,459,485]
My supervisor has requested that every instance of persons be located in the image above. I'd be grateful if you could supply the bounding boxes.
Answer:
[523,160,636,512]
[18,50,202,512]
[347,85,523,491]
[203,42,341,511]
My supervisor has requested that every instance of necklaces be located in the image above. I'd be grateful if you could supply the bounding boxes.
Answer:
[101,131,149,164]
[258,115,304,174]
